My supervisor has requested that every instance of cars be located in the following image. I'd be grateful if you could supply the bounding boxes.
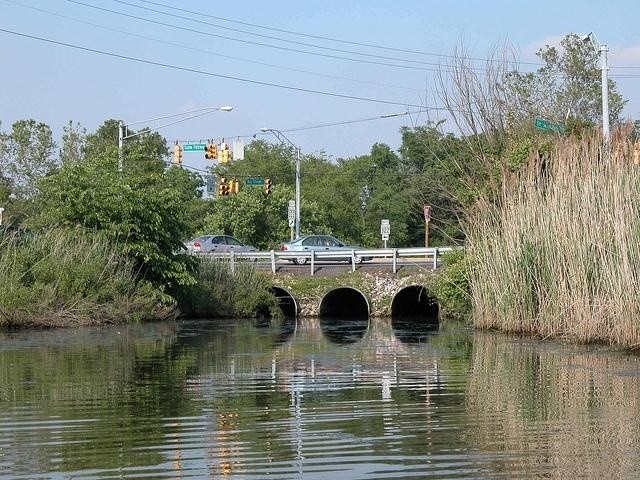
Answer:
[279,234,374,265]
[181,352,274,398]
[272,354,362,391]
[178,235,260,262]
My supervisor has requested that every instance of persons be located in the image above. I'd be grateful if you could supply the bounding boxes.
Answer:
[322,239,332,245]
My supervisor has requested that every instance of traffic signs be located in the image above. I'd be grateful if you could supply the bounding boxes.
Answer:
[183,144,206,152]
[245,178,264,186]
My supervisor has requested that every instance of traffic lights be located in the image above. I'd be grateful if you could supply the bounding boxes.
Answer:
[210,144,216,160]
[221,178,228,184]
[204,143,211,159]
[218,184,225,200]
[264,177,273,196]
[174,145,183,164]
[224,184,230,197]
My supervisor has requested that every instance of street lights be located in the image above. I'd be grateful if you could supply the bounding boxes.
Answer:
[532,118,567,133]
[582,31,609,152]
[118,106,233,172]
[261,128,300,239]
